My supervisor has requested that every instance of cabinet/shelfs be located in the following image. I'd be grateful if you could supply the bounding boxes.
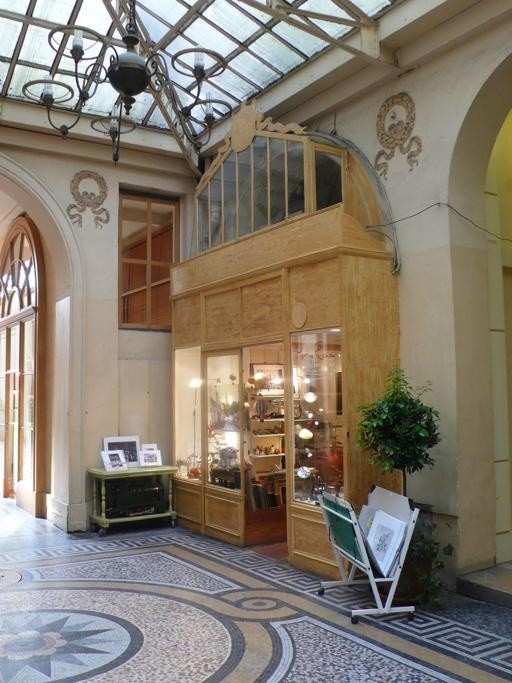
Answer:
[248,393,288,459]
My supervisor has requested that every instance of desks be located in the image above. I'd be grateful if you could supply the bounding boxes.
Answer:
[86,462,180,537]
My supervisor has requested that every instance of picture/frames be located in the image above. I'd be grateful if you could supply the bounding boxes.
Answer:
[249,362,289,397]
[100,434,162,473]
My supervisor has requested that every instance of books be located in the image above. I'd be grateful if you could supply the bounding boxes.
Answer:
[355,503,408,576]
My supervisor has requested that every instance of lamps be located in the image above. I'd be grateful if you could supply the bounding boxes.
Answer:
[20,0,236,166]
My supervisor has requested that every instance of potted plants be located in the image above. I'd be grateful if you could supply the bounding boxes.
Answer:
[353,363,456,611]
